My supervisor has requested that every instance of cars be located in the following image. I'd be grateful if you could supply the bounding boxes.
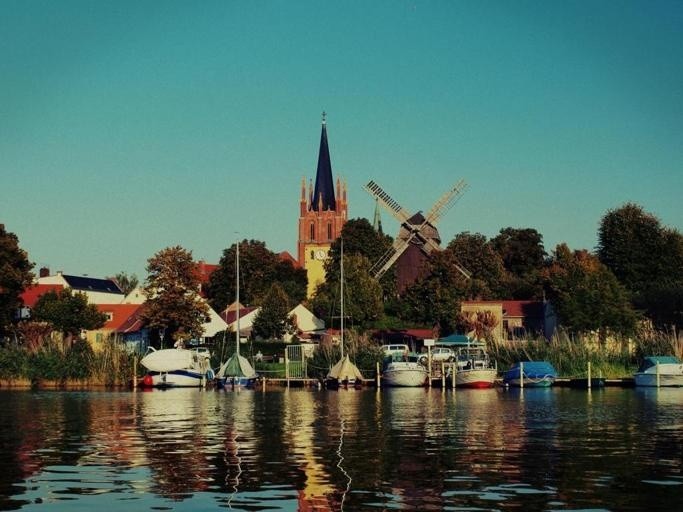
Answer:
[192,346,212,361]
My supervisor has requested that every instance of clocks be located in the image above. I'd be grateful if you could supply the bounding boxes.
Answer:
[315,249,325,261]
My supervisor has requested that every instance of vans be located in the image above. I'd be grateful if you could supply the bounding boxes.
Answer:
[378,344,410,361]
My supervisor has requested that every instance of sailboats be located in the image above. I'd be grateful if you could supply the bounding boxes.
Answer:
[324,237,366,388]
[214,239,258,387]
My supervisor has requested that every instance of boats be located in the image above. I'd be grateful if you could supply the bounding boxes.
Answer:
[139,349,214,388]
[633,355,683,387]
[447,337,497,389]
[502,360,558,388]
[383,352,426,386]
[569,376,612,391]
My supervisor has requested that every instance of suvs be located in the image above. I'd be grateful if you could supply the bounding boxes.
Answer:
[414,346,456,363]
